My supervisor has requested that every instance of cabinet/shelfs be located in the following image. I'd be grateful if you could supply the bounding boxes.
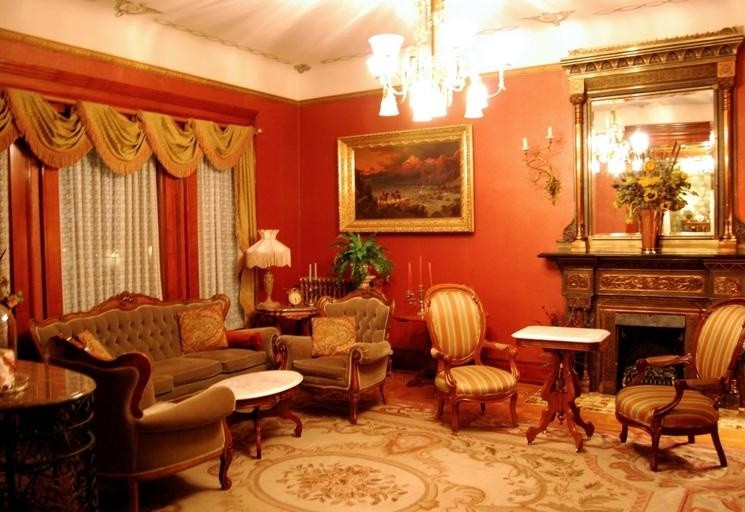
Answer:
[536,251,745,407]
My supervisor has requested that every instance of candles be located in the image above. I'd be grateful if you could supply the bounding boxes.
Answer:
[547,127,554,138]
[308,261,312,286]
[523,136,529,150]
[418,256,424,287]
[313,261,316,285]
[429,268,434,289]
[407,262,412,295]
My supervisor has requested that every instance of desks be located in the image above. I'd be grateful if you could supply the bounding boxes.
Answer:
[258,307,317,335]
[510,325,613,451]
[0,357,101,512]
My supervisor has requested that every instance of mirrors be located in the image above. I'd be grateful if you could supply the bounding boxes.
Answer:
[587,87,718,239]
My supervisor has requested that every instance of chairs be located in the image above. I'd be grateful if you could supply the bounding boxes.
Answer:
[615,299,745,471]
[423,282,520,433]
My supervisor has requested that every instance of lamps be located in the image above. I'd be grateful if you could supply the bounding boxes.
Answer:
[364,0,511,121]
[245,227,292,309]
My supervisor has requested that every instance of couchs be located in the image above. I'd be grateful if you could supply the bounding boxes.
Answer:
[20,290,290,406]
[275,287,395,423]
[47,334,236,512]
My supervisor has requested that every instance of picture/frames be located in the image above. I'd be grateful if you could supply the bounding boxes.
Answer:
[334,123,475,238]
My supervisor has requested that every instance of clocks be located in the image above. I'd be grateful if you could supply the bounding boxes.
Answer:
[285,284,301,307]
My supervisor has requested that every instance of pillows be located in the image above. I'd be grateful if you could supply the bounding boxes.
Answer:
[308,313,357,356]
[178,304,227,351]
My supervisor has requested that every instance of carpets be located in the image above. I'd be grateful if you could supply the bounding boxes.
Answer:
[127,398,745,512]
[523,380,745,429]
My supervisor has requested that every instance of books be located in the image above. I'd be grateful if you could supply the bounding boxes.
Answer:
[301,277,359,305]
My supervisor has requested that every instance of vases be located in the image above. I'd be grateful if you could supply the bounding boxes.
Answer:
[637,206,660,255]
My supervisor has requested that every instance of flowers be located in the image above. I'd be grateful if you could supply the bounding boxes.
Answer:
[611,158,692,218]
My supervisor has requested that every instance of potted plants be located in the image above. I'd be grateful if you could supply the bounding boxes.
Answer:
[0,251,30,396]
[330,234,393,289]
